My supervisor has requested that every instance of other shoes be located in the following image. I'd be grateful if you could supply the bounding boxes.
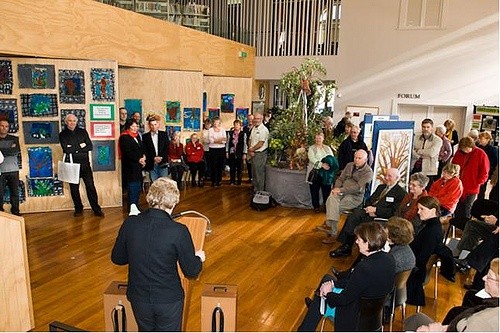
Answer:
[74,207,84,216]
[94,210,104,217]
[237,180,241,185]
[198,181,203,187]
[247,179,252,184]
[212,182,215,187]
[216,181,219,186]
[439,271,456,282]
[316,224,332,233]
[455,262,471,272]
[315,207,321,212]
[191,181,197,187]
[458,251,472,264]
[322,236,336,243]
[464,285,477,289]
[229,180,235,185]
[12,212,22,216]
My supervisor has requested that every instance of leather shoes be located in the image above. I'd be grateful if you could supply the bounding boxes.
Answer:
[305,297,313,309]
[332,268,345,280]
[329,245,353,258]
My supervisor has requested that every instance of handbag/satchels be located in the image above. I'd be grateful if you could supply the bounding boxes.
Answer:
[308,161,323,184]
[58,153,81,185]
[249,190,272,211]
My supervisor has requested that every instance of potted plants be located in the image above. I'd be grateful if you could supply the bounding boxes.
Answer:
[265,54,346,209]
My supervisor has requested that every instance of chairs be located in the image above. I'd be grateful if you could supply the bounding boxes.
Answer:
[320,191,456,333]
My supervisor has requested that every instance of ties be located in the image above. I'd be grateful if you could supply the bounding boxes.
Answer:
[377,186,390,201]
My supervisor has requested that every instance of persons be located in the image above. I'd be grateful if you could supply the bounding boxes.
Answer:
[203,113,269,191]
[59,114,104,216]
[168,131,183,190]
[119,108,169,214]
[185,134,205,186]
[295,112,499,332]
[0,120,21,216]
[111,177,206,332]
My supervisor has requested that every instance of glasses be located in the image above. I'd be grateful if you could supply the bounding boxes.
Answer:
[487,270,499,283]
